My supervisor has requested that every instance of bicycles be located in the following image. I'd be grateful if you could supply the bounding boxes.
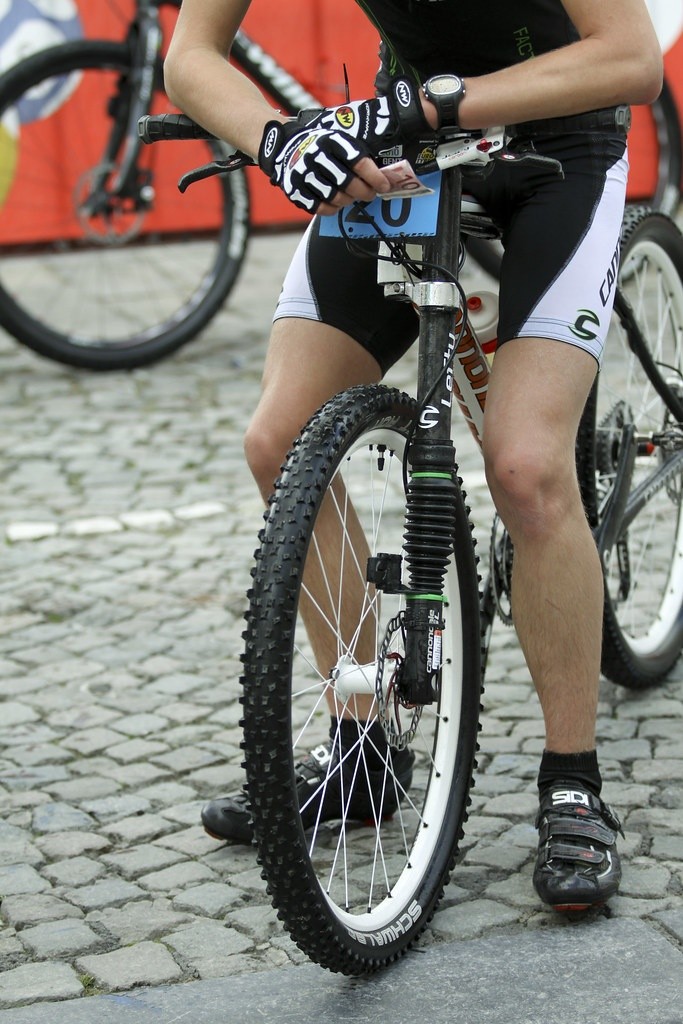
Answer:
[137,114,683,978]
[1,0,683,372]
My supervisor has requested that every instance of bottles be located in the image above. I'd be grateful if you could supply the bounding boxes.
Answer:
[466,290,497,368]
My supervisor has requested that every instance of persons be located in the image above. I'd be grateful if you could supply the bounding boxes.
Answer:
[161,0,664,914]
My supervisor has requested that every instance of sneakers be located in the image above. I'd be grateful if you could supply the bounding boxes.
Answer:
[201,738,415,843]
[534,778,624,912]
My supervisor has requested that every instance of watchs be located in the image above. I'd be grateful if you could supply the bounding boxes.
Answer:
[421,74,466,135]
